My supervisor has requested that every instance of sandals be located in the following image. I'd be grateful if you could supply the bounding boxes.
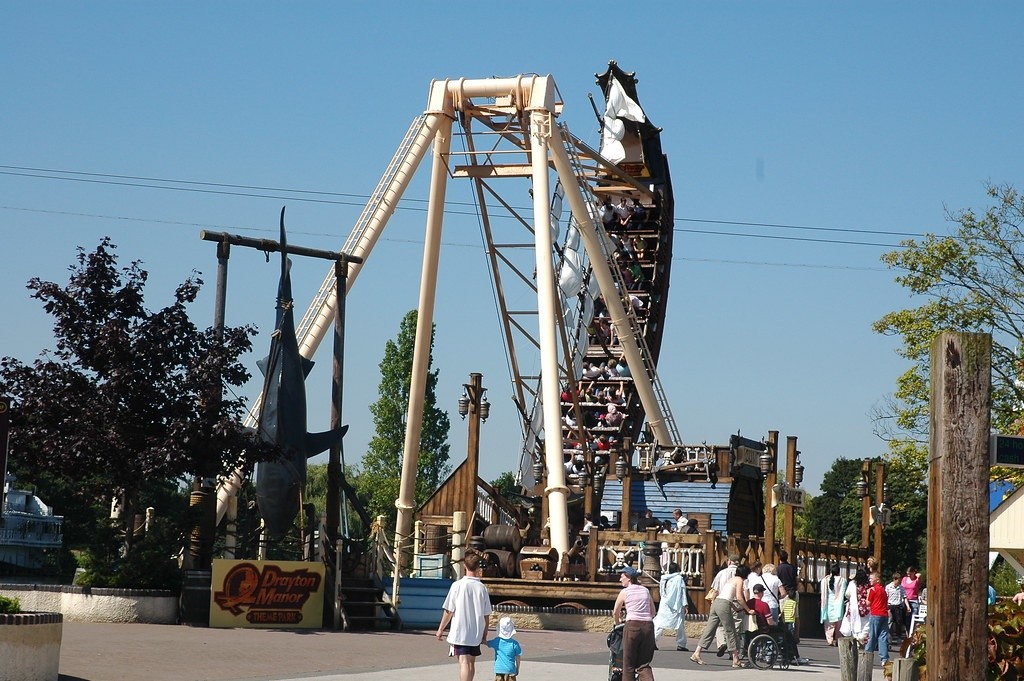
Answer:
[732,660,747,668]
[690,654,706,665]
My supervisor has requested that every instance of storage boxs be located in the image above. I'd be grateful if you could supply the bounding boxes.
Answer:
[416,553,449,579]
[518,545,559,580]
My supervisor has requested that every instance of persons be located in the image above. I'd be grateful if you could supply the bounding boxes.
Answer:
[613,566,658,681]
[988,585,996,605]
[652,562,691,652]
[579,509,704,574]
[820,556,921,666]
[561,380,629,478]
[435,554,523,681]
[1013,583,1024,607]
[921,587,928,605]
[689,551,812,668]
[579,192,652,378]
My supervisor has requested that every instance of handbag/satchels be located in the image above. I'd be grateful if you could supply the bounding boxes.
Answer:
[705,588,715,600]
[839,617,851,637]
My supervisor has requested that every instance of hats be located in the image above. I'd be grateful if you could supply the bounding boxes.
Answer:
[496,617,516,639]
[616,567,638,578]
[753,584,766,593]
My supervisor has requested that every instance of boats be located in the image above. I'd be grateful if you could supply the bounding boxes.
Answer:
[509,57,678,578]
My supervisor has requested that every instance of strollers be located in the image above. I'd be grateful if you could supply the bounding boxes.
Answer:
[605,618,640,681]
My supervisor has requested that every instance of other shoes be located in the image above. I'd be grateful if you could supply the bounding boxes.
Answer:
[654,644,659,650]
[717,644,727,657]
[677,646,689,651]
[882,661,886,666]
[898,640,902,649]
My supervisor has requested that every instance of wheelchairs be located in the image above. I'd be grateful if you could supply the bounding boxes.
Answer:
[736,608,793,670]
